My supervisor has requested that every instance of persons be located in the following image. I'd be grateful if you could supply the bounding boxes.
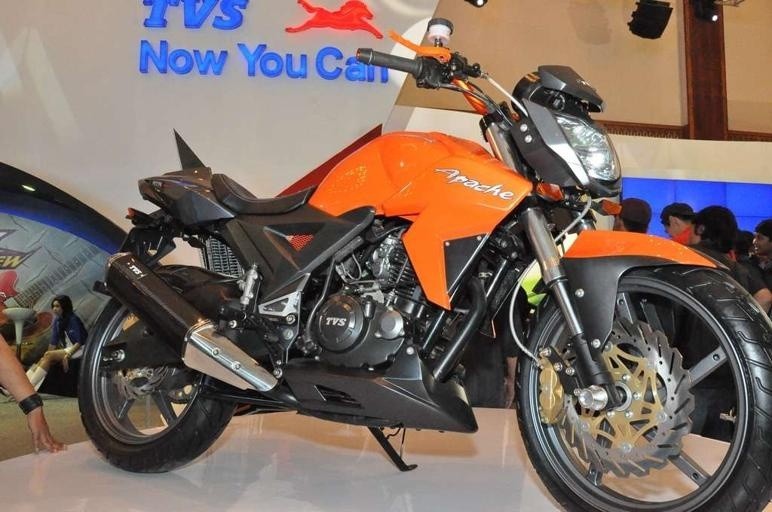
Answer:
[619,197,653,319]
[0,295,87,403]
[661,202,771,432]
[464,284,529,409]
[0,333,68,454]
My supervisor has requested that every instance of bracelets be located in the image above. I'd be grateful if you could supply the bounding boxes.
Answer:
[18,394,43,415]
[64,351,72,357]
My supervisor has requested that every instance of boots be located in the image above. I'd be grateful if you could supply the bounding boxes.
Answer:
[0,363,48,402]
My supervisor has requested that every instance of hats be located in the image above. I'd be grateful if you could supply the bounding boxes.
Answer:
[621,196,736,225]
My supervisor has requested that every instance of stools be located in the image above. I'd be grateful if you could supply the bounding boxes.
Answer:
[2,308,33,363]
[22,358,80,397]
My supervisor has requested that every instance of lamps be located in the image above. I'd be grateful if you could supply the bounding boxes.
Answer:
[689,0,718,24]
[464,0,487,8]
[627,1,673,40]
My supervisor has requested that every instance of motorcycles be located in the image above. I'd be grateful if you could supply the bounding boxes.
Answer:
[73,17,771,509]
